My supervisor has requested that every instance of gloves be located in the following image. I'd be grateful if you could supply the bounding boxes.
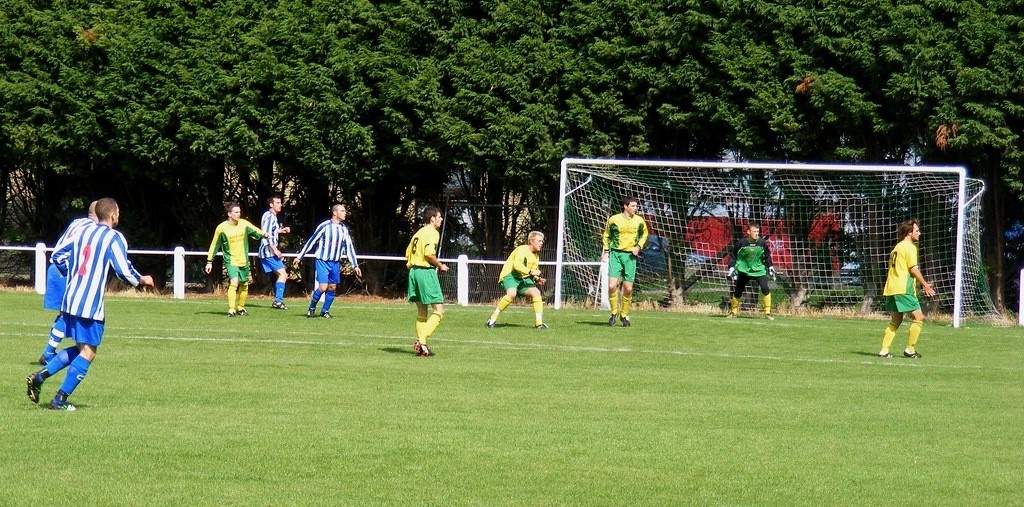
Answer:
[727,267,737,280]
[769,266,777,281]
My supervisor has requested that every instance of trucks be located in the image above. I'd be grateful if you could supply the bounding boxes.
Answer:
[841,261,863,285]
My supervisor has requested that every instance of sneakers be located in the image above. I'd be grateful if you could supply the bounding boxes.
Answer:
[320,312,331,317]
[237,308,248,315]
[878,353,893,358]
[487,318,496,327]
[50,395,77,411]
[26,371,42,403]
[307,308,315,318]
[414,341,435,357]
[272,302,288,310]
[903,350,922,359]
[227,310,235,318]
[536,323,548,329]
[620,317,630,327]
[609,314,617,326]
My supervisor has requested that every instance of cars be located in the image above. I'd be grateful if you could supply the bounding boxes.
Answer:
[636,235,712,279]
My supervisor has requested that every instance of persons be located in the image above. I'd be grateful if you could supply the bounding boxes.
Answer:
[601,196,649,327]
[26,198,154,411]
[205,196,291,317]
[292,202,362,318]
[406,206,449,357]
[879,218,935,360]
[727,223,776,321]
[486,231,550,331]
